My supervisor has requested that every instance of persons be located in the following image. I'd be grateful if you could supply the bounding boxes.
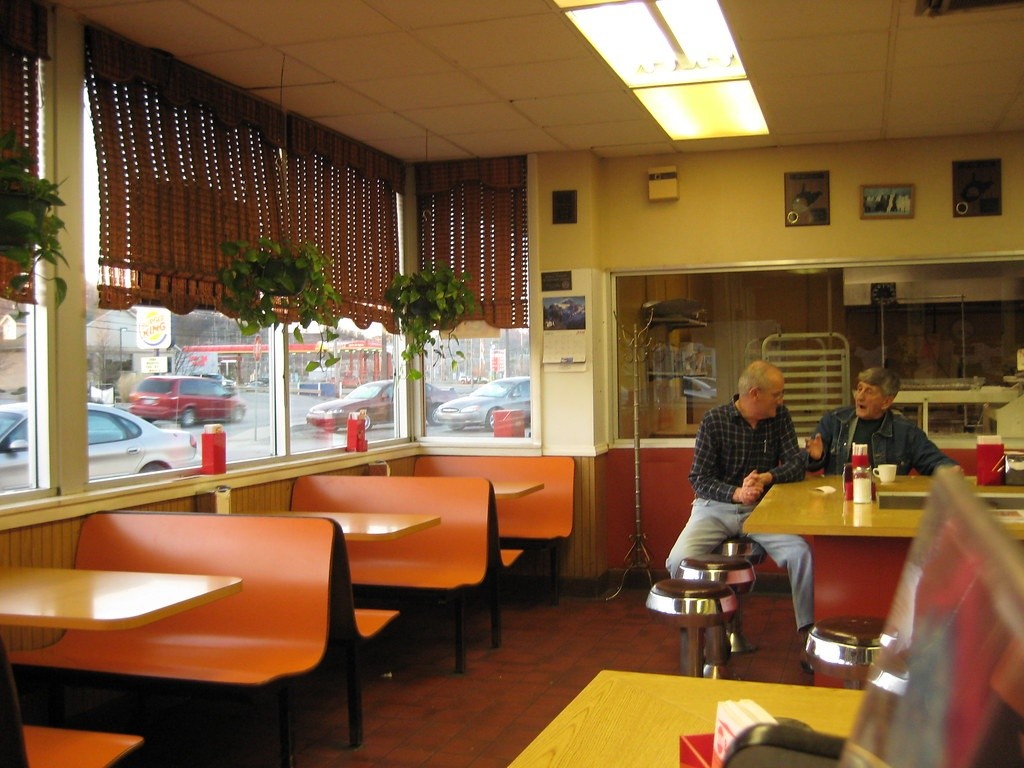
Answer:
[665,361,815,672]
[802,366,964,478]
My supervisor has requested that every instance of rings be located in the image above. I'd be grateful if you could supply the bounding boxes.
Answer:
[748,492,750,495]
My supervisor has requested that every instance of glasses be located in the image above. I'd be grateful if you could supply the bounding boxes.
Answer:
[750,387,785,400]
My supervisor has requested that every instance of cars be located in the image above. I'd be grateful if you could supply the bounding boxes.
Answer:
[128,375,247,428]
[195,373,239,397]
[433,376,530,432]
[0,403,197,493]
[307,379,452,432]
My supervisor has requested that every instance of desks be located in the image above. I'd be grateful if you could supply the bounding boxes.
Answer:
[852,390,1019,437]
[507,670,866,768]
[742,475,1024,538]
[230,480,545,542]
[0,566,242,631]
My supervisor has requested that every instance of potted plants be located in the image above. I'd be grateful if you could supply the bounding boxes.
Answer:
[217,235,344,372]
[0,127,69,310]
[385,258,483,380]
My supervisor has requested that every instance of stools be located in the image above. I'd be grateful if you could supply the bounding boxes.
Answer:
[645,536,767,678]
[805,616,886,689]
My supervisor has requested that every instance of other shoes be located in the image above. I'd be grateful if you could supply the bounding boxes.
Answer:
[800,648,814,675]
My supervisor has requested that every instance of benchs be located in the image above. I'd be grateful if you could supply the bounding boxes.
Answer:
[0,455,575,768]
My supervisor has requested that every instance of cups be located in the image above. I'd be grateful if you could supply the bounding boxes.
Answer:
[852,455,869,469]
[872,464,898,483]
[977,444,1004,486]
[853,467,872,503]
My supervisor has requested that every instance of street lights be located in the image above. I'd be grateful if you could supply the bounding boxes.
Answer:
[119,326,127,375]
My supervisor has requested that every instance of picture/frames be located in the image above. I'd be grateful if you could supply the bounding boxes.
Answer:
[858,183,915,220]
